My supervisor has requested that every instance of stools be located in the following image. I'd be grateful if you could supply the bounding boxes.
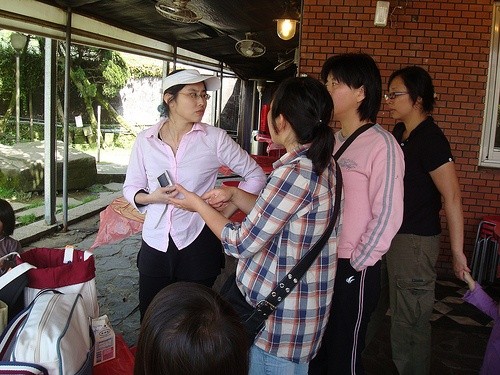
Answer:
[470,217,500,290]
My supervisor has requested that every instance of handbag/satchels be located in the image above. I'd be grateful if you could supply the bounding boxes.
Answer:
[219,273,265,344]
[1,288,93,375]
[0,245,98,325]
[0,300,8,337]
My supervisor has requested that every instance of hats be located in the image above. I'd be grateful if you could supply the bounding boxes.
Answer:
[163,69,221,93]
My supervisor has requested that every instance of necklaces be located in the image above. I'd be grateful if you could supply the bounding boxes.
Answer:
[167,123,180,150]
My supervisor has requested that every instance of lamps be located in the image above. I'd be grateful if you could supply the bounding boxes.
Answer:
[273,0,302,40]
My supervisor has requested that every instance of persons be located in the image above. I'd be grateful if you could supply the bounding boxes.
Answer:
[122,52,500,375]
[0,198,23,276]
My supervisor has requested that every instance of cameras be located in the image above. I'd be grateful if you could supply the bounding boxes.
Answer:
[156,170,174,194]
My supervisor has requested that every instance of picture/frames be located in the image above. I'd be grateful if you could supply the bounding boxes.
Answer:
[478,0,500,168]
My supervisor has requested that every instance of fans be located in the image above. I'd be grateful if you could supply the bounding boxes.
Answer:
[235,31,265,57]
[154,0,203,23]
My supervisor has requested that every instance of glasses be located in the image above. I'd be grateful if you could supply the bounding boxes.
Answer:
[176,93,211,100]
[384,92,409,100]
